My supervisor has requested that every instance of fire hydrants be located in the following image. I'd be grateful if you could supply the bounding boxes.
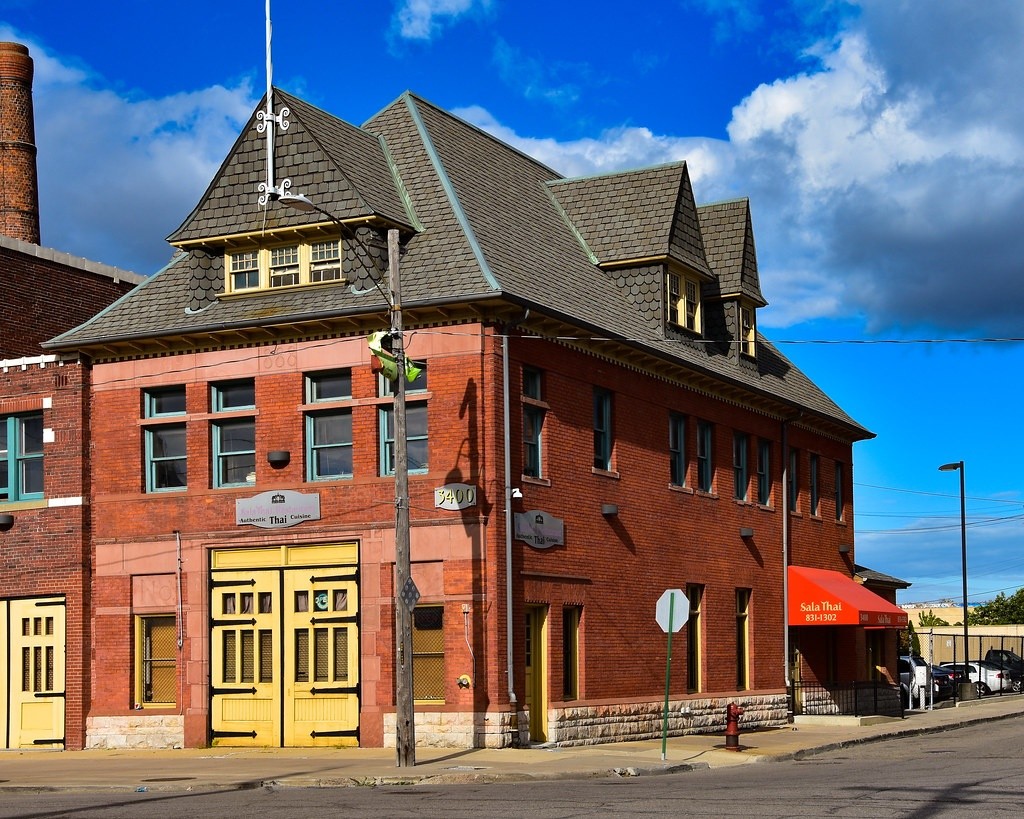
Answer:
[723,701,744,750]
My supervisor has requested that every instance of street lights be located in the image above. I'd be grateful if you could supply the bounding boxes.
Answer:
[940,460,972,681]
[280,195,419,770]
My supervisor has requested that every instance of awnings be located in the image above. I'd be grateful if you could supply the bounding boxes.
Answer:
[787,565,908,628]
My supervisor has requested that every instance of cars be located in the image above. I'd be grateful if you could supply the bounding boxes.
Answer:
[940,660,1013,697]
[985,649,1024,693]
[899,654,938,709]
[927,663,967,701]
